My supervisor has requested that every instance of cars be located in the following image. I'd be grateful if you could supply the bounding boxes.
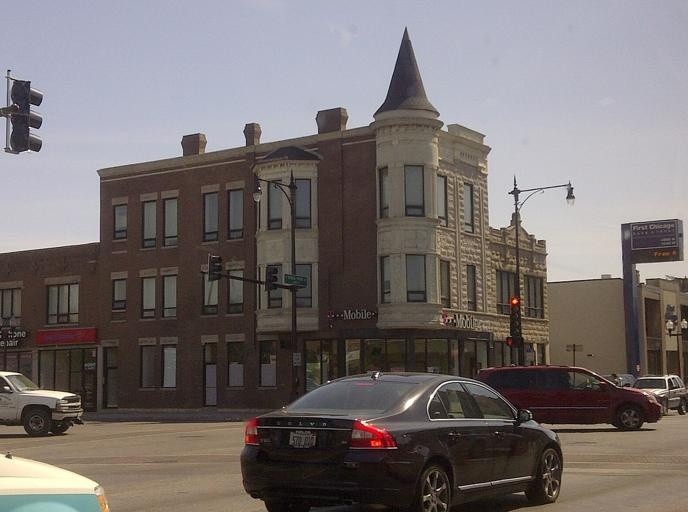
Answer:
[240,371,563,512]
[0,452,110,512]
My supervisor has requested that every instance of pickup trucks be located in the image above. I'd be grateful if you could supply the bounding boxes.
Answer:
[0,371,84,436]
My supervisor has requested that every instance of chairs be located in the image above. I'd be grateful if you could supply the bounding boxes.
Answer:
[429,391,451,418]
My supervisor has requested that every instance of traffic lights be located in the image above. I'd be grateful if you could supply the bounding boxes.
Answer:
[209,252,225,288]
[263,262,281,296]
[0,69,43,155]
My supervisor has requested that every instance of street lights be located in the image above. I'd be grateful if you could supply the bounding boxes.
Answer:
[664,320,687,380]
[508,173,575,366]
[207,168,306,405]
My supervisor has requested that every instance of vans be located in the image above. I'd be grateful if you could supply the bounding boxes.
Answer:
[477,366,687,431]
